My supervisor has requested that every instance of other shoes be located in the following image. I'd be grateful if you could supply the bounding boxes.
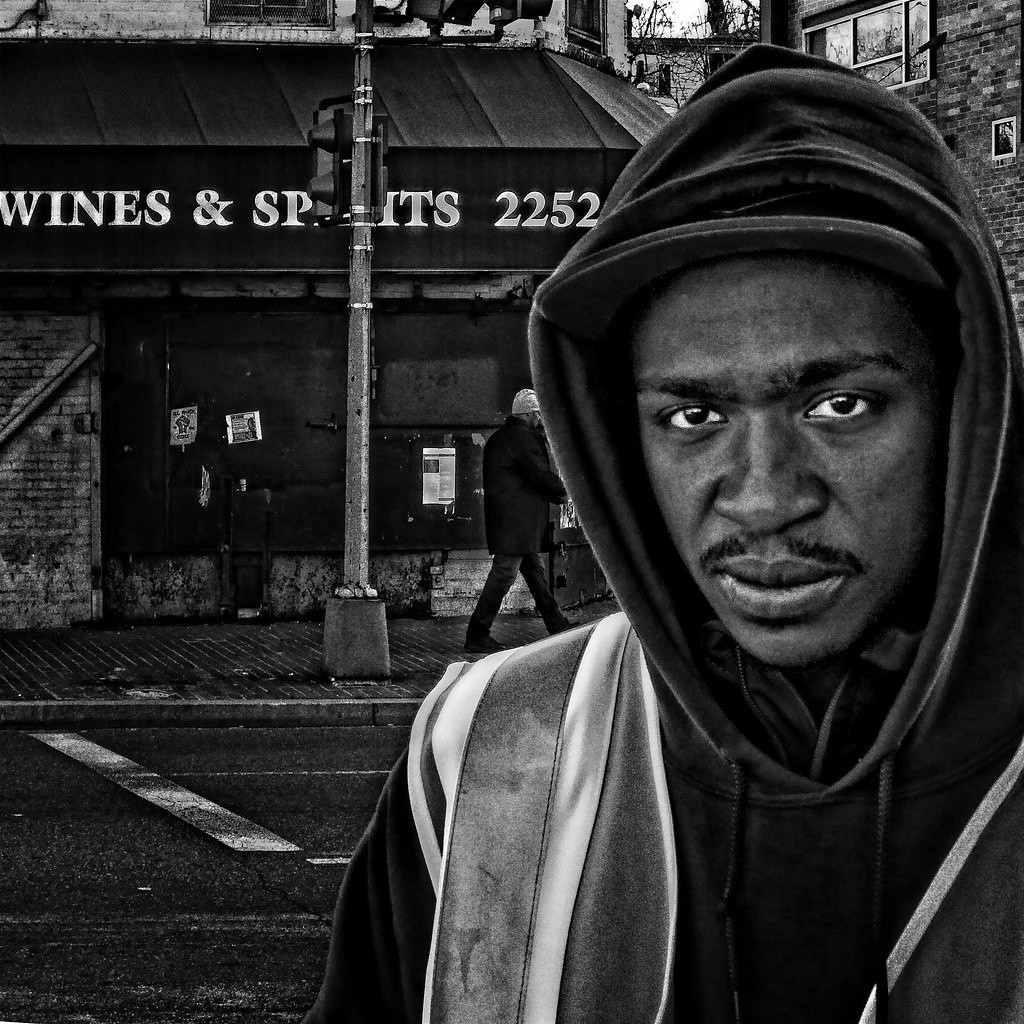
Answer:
[552,622,580,635]
[464,639,506,653]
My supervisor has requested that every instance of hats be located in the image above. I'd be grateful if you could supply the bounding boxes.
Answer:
[512,389,540,414]
[535,186,956,329]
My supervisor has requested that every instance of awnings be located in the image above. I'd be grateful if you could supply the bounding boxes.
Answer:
[0,36,675,277]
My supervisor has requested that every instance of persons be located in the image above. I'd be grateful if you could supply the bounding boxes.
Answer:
[465,388,582,653]
[307,46,1024,1024]
